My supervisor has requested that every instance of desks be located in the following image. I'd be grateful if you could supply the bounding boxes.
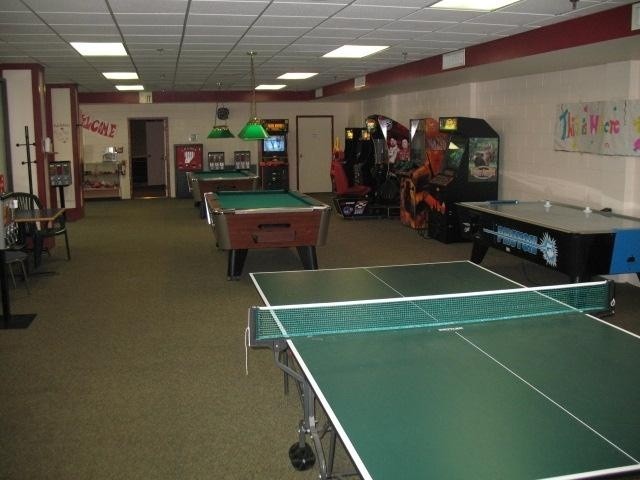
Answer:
[13,208,71,268]
[248,260,638,478]
[455,199,638,312]
[203,188,333,281]
[184,169,259,219]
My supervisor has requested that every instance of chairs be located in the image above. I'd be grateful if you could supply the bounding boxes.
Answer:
[329,160,370,216]
[5,250,33,295]
[8,192,71,268]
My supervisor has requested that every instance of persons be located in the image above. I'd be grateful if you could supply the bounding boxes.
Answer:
[396,138,411,161]
[387,136,401,163]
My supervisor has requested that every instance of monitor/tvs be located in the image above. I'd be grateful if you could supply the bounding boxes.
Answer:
[262,134,287,156]
[446,142,466,172]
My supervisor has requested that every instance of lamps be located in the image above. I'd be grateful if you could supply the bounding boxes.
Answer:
[207,81,235,138]
[47,160,72,208]
[44,137,52,153]
[238,50,271,139]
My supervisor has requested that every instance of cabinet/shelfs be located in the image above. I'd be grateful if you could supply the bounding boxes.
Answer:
[81,161,122,199]
